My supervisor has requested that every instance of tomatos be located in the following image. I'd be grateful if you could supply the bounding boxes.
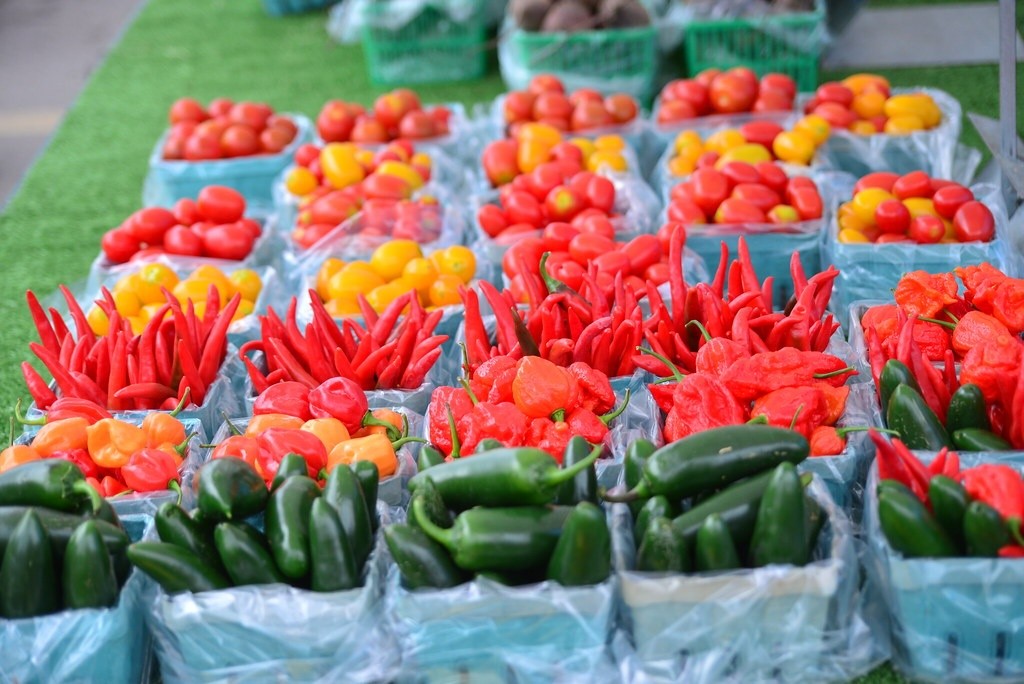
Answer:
[88,74,997,328]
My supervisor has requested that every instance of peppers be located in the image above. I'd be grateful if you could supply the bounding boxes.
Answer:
[0,221,1024,623]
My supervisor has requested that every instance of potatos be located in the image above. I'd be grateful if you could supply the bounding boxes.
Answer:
[507,0,651,35]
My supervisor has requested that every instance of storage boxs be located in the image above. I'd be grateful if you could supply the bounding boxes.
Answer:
[0,89,1024,684]
